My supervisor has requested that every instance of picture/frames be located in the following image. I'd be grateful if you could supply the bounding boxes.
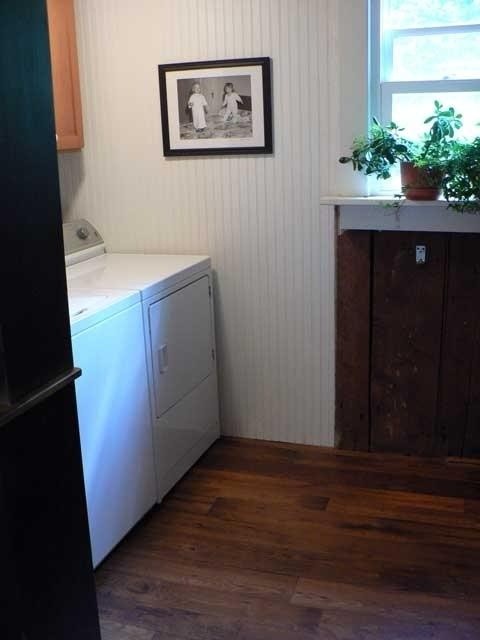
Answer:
[157,57,273,158]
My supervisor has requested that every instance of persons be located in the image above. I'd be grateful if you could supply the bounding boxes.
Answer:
[221,81,244,127]
[187,82,210,132]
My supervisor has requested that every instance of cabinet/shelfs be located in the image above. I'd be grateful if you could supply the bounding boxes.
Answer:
[46,1,85,151]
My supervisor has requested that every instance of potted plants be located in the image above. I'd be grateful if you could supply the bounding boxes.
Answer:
[338,99,480,221]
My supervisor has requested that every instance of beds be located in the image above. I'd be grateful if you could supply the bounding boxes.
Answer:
[179,94,253,140]
